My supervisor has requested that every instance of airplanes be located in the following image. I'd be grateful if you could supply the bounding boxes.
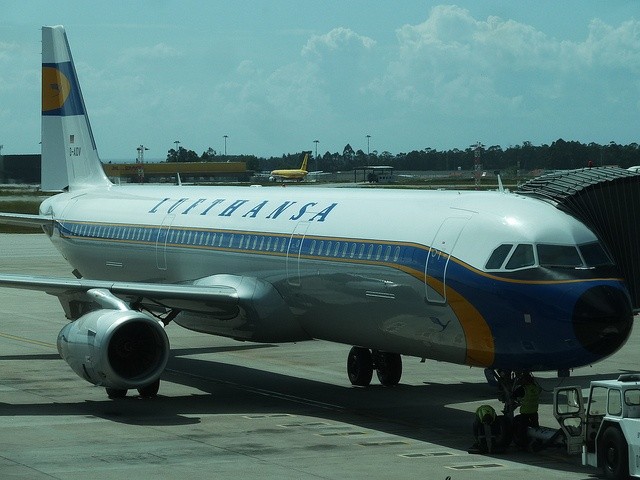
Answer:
[0,25,633,453]
[269,154,308,183]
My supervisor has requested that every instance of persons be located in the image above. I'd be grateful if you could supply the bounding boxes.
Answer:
[473,405,498,452]
[513,374,543,449]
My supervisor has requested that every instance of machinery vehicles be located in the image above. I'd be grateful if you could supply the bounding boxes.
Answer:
[554,370,640,480]
